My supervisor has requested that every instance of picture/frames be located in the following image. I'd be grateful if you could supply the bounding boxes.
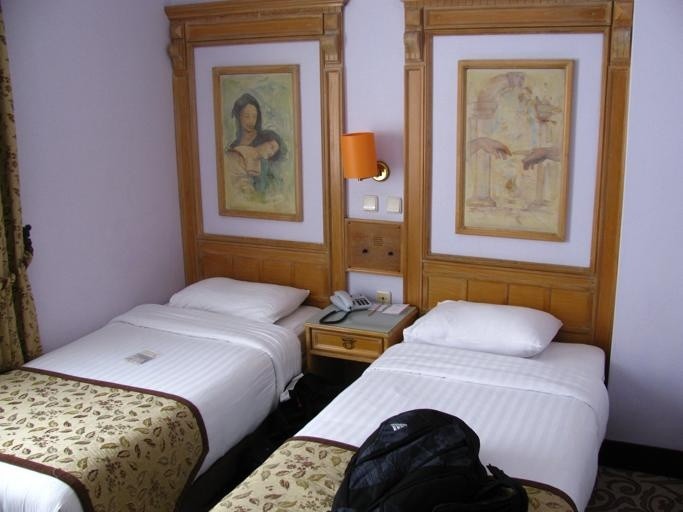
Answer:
[212,63,306,224]
[453,57,576,244]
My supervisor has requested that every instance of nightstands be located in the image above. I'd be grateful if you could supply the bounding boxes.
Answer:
[302,302,419,373]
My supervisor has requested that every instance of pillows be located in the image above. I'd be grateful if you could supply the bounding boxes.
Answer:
[167,276,310,324]
[401,300,565,358]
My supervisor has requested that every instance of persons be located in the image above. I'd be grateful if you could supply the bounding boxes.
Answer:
[228,129,288,196]
[230,94,261,149]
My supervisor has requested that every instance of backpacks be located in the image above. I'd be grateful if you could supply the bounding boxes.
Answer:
[329,407,530,512]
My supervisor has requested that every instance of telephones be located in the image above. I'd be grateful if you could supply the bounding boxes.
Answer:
[317,288,371,326]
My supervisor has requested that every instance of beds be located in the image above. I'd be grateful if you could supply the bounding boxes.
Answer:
[210,340,610,511]
[1,302,326,512]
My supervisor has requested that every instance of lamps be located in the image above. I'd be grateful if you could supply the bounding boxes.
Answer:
[340,130,390,182]
